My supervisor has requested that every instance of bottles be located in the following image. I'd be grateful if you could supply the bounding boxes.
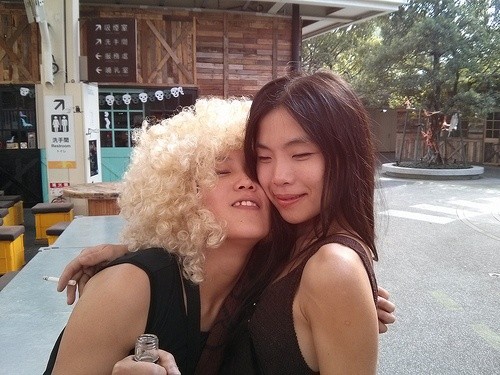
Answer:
[134,334,160,365]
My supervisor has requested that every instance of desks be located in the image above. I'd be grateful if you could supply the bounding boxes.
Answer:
[64,182,125,216]
[1,215,132,375]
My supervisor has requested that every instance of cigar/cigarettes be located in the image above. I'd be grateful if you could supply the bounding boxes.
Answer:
[43,276,75,286]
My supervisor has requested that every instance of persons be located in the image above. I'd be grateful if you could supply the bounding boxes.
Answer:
[44,96,396,375]
[56,69,378,375]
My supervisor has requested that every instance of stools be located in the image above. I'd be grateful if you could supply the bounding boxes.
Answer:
[0,190,24,226]
[46,223,70,246]
[32,203,74,245]
[0,226,26,275]
